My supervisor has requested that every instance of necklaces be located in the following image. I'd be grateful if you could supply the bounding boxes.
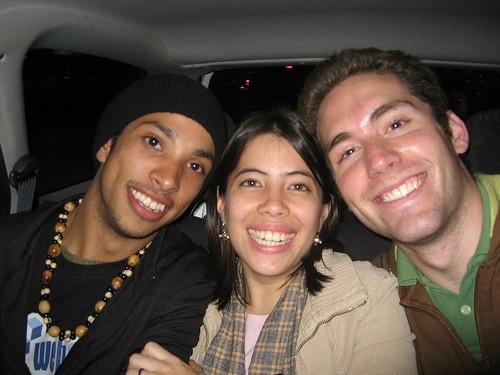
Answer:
[38,198,152,343]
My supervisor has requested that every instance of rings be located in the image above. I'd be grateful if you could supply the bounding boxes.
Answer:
[138,368,144,375]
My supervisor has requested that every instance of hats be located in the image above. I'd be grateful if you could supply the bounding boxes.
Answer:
[93,73,227,167]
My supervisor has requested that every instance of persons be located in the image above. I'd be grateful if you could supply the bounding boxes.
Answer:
[125,104,418,375]
[0,73,227,375]
[300,48,500,375]
